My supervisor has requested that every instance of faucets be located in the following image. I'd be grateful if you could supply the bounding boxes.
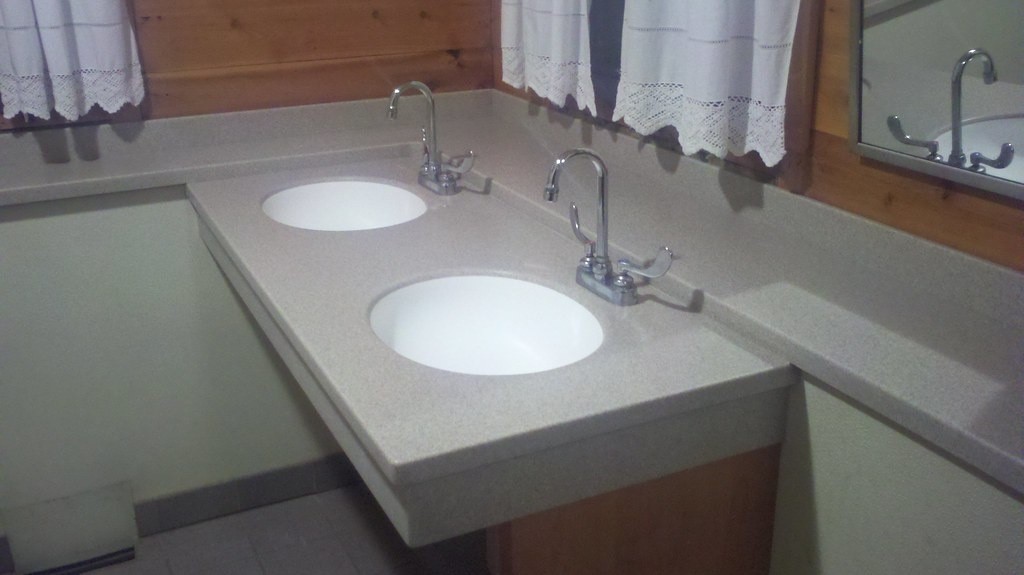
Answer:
[387,79,443,192]
[543,145,614,295]
[944,46,1001,168]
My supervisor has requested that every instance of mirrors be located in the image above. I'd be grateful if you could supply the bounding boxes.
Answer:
[842,0,1024,206]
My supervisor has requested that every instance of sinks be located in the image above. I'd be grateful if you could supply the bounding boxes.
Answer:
[929,104,1024,190]
[364,267,610,385]
[257,172,432,235]
